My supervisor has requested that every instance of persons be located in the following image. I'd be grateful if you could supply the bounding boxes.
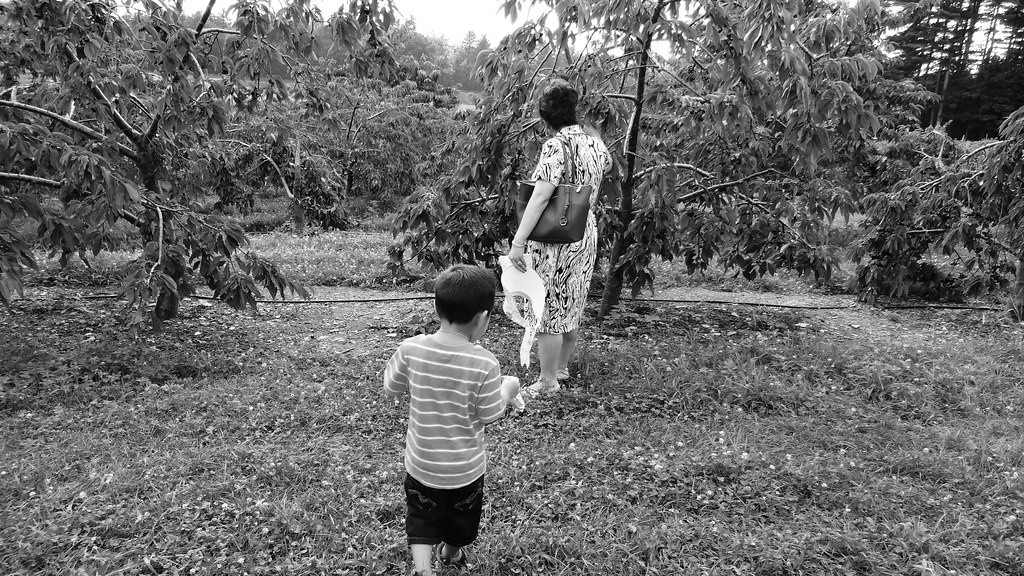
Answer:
[510,80,612,390]
[383,266,520,576]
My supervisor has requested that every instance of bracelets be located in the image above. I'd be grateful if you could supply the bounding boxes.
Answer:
[511,241,526,247]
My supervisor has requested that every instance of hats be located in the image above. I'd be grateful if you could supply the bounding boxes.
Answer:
[500,265,545,369]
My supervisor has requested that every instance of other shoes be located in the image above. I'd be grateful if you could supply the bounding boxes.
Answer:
[556,368,570,379]
[437,543,467,571]
[522,383,560,396]
[412,569,437,576]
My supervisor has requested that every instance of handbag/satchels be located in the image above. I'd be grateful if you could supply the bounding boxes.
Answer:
[515,135,592,244]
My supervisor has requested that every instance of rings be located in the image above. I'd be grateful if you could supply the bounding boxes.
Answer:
[517,261,521,265]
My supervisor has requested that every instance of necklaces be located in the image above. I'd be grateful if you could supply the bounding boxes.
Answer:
[440,328,470,342]
